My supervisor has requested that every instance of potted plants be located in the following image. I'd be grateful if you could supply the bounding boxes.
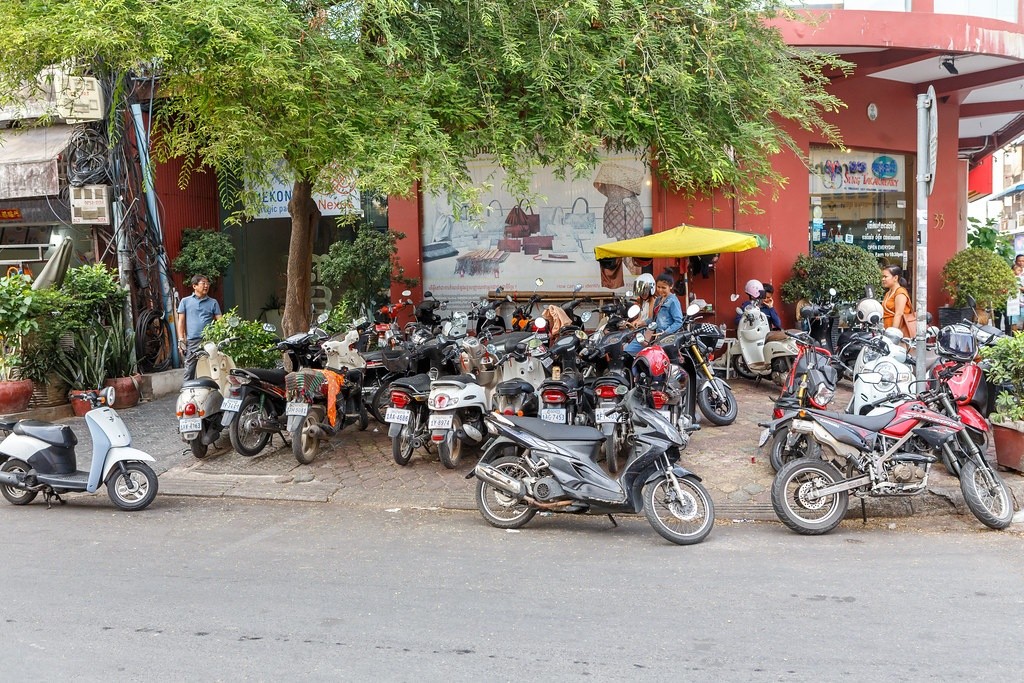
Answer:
[0,262,147,417]
[256,290,285,340]
[937,245,1019,331]
[978,329,1024,476]
[779,239,882,355]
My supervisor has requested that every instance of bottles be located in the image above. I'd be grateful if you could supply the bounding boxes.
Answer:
[720,320,727,338]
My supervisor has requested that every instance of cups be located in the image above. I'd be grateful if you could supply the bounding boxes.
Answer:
[707,304,713,310]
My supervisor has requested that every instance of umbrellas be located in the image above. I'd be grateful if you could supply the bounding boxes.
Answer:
[592,223,766,330]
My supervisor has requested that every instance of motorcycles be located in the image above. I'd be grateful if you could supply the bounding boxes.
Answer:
[175,275,1012,483]
[466,370,717,546]
[769,362,1013,537]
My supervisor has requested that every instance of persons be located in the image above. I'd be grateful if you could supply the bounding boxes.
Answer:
[606,273,683,357]
[994,255,1024,335]
[735,283,780,327]
[176,275,222,382]
[880,266,914,350]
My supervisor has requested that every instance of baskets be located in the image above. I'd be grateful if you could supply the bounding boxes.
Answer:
[381,349,410,372]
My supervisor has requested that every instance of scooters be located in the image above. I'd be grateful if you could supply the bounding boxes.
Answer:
[0,385,159,511]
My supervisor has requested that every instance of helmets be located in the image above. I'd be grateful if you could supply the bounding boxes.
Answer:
[856,299,885,325]
[935,325,977,363]
[745,279,766,299]
[631,345,672,382]
[632,273,657,295]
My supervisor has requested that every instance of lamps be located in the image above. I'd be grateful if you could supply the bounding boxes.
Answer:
[942,56,958,75]
